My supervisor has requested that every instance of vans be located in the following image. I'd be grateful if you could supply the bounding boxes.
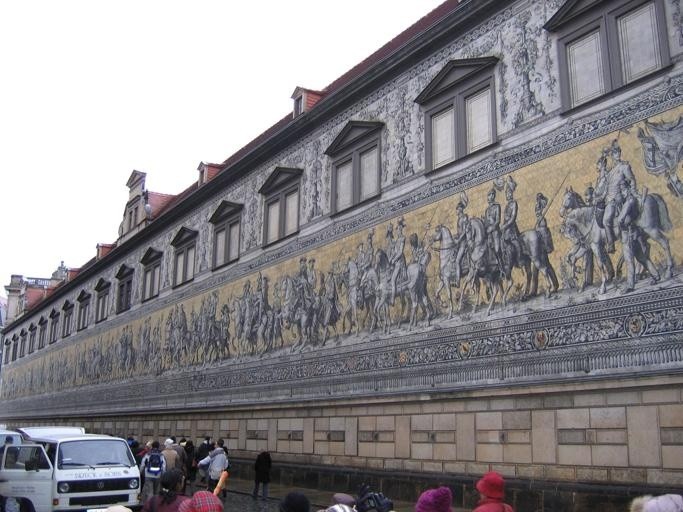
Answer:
[0,422,24,468]
[0,425,141,512]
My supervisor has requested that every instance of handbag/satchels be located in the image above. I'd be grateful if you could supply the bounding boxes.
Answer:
[191,457,197,468]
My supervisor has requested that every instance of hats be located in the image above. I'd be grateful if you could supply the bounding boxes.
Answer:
[178,491,225,512]
[317,504,358,512]
[475,471,506,499]
[164,439,174,445]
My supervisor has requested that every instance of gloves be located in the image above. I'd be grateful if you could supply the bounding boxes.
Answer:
[371,492,393,511]
[356,483,375,512]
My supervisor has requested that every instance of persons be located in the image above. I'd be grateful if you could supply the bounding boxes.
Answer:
[472,472,514,512]
[144,467,225,511]
[630,494,683,512]
[126,435,228,497]
[252,447,272,501]
[327,479,394,511]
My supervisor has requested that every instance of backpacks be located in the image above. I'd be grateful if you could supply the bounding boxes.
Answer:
[146,453,163,476]
